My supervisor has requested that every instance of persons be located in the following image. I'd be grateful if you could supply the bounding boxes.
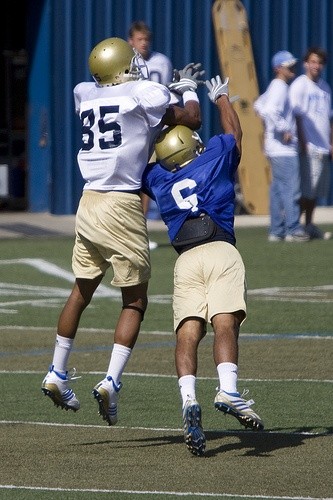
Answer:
[252,51,312,243]
[288,50,333,241]
[127,19,177,252]
[142,75,264,457]
[39,36,207,427]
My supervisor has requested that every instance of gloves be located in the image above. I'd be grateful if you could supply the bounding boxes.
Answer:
[205,75,230,103]
[167,63,206,94]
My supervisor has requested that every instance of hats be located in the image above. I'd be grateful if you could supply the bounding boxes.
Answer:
[272,50,298,68]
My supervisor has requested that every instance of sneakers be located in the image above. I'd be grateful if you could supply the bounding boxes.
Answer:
[182,394,207,458]
[213,386,263,431]
[92,374,123,426]
[41,364,81,412]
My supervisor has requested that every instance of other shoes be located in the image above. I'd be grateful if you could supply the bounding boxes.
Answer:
[268,233,285,241]
[286,230,310,242]
[305,225,320,235]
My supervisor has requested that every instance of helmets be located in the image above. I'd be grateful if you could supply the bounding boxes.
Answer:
[88,36,138,88]
[155,121,204,172]
